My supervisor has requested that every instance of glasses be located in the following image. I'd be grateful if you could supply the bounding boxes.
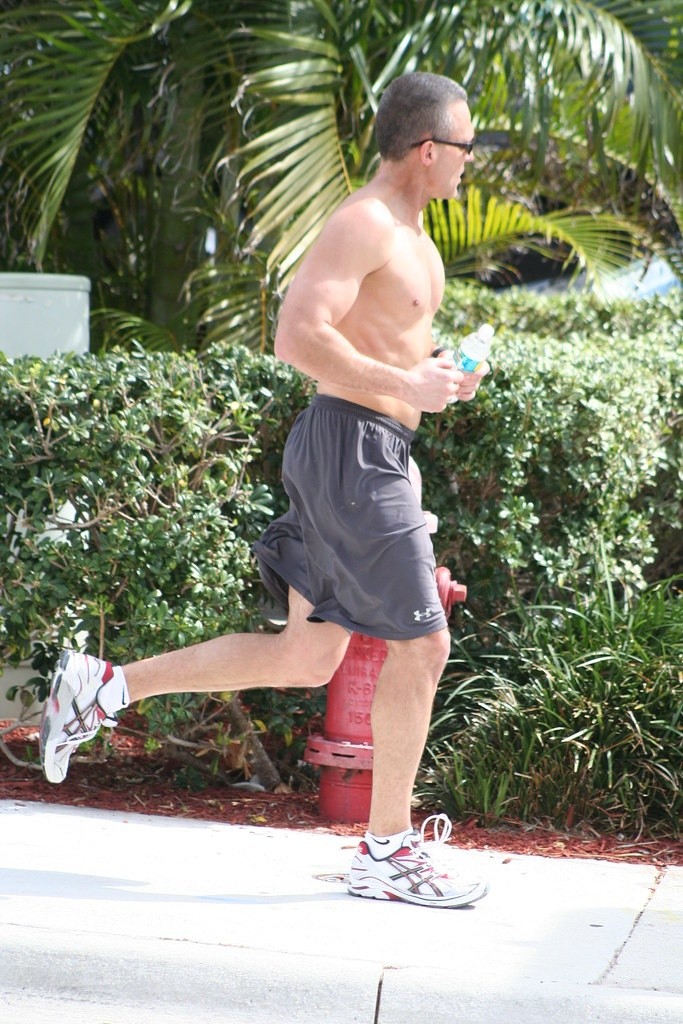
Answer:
[412,137,476,154]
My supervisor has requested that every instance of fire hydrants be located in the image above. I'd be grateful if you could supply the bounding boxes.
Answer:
[301,511,469,817]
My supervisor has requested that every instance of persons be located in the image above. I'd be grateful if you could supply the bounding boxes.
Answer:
[42,72,491,908]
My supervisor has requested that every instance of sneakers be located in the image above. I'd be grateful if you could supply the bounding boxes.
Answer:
[348,814,488,908]
[40,649,118,784]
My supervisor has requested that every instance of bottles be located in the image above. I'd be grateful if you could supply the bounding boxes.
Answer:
[448,323,495,405]
